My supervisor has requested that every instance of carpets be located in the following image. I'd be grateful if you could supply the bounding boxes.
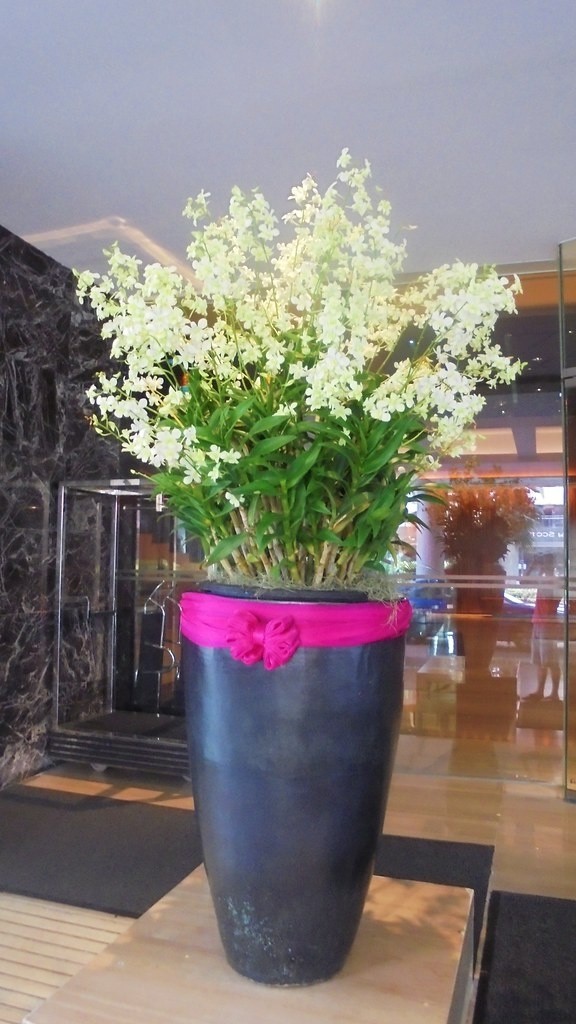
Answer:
[0,783,576,1024]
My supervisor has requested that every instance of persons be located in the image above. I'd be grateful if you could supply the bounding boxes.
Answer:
[519,551,567,708]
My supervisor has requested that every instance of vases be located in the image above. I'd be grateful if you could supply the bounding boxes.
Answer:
[179,580,412,986]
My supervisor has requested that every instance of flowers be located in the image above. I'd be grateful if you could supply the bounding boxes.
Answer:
[420,458,539,567]
[70,147,530,578]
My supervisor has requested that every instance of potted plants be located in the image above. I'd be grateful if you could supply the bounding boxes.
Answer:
[445,566,507,614]
